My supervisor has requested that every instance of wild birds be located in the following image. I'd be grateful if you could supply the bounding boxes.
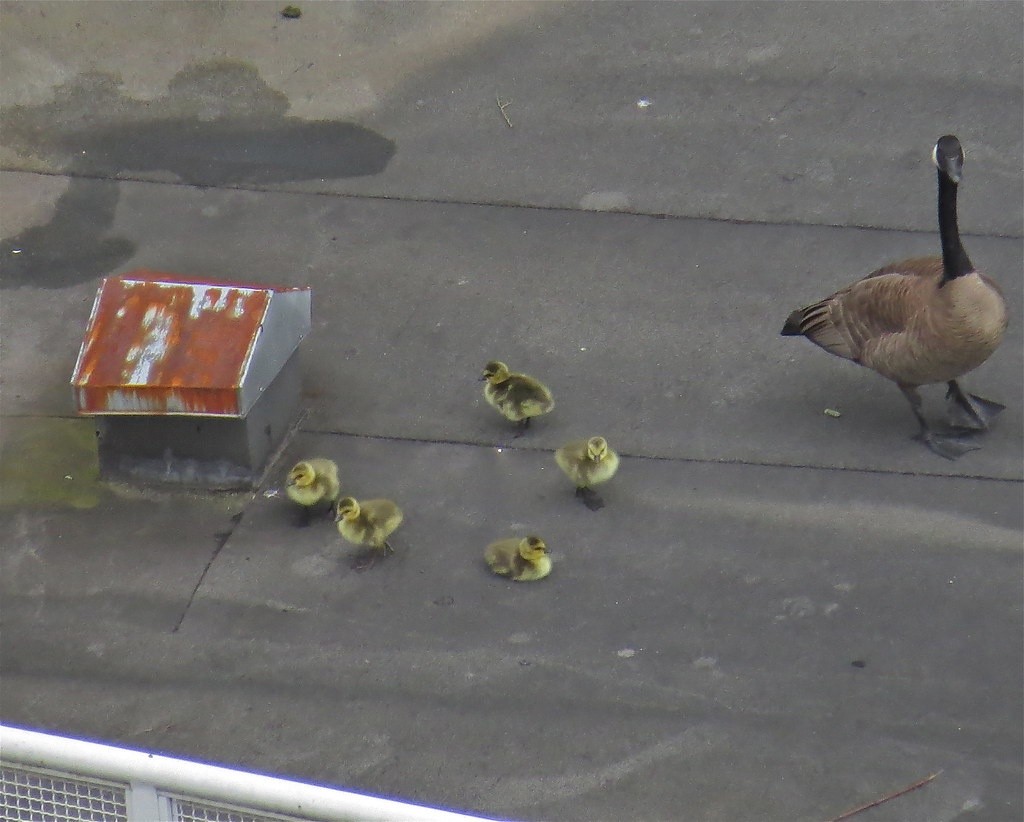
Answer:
[285,459,340,528]
[554,437,621,512]
[334,496,402,569]
[478,361,554,433]
[780,134,1007,462]
[484,536,553,581]
